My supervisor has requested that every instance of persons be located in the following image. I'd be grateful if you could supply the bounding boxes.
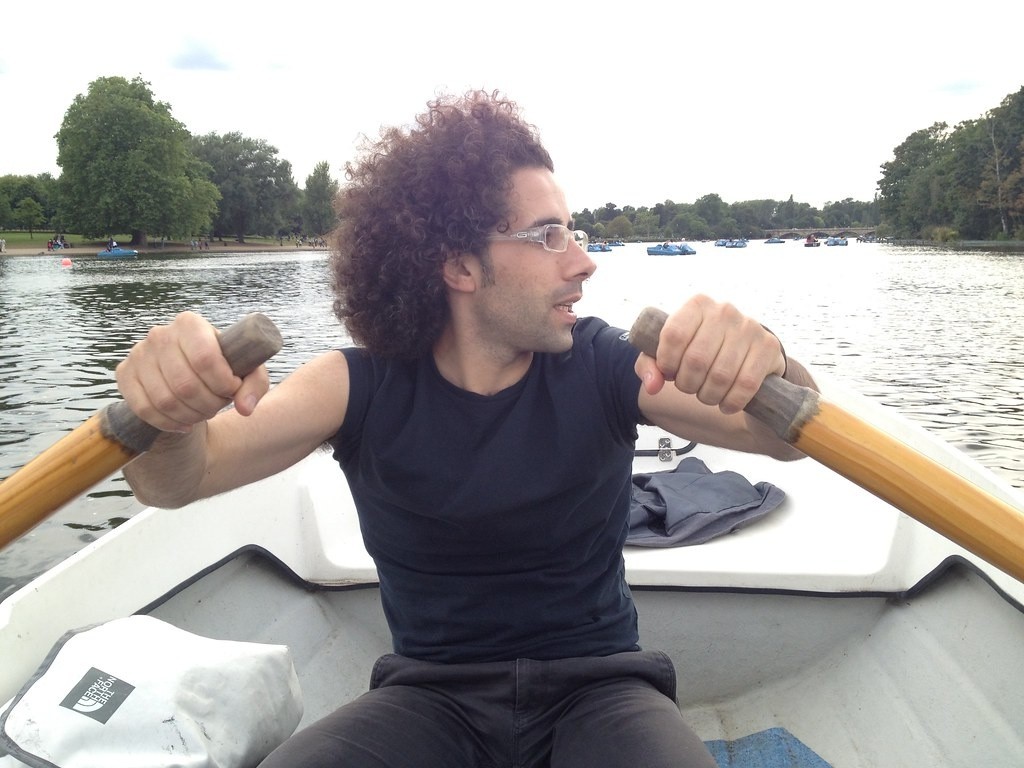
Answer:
[279,232,328,249]
[601,241,607,248]
[1,236,7,253]
[729,236,733,242]
[46,234,66,251]
[679,237,687,249]
[104,237,118,253]
[104,91,824,768]
[153,235,166,249]
[663,238,673,248]
[806,233,847,243]
[188,238,209,251]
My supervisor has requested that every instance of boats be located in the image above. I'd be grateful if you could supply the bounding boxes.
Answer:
[588,244,612,252]
[0,423,1021,768]
[715,241,747,247]
[609,242,625,246]
[98,248,138,257]
[827,238,848,246]
[647,246,696,256]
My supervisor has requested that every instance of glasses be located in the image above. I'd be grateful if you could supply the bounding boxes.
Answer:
[485,224,590,257]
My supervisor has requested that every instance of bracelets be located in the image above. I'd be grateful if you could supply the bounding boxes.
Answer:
[759,323,787,379]
